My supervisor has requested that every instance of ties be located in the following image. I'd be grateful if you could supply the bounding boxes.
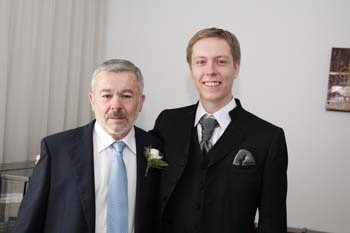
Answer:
[107,141,129,233]
[199,116,218,154]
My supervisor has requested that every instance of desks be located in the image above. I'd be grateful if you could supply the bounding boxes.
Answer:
[0,158,38,205]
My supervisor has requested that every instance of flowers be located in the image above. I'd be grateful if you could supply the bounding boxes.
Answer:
[143,145,168,176]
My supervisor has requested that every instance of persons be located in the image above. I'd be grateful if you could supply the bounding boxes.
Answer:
[36,27,288,233]
[12,59,166,233]
